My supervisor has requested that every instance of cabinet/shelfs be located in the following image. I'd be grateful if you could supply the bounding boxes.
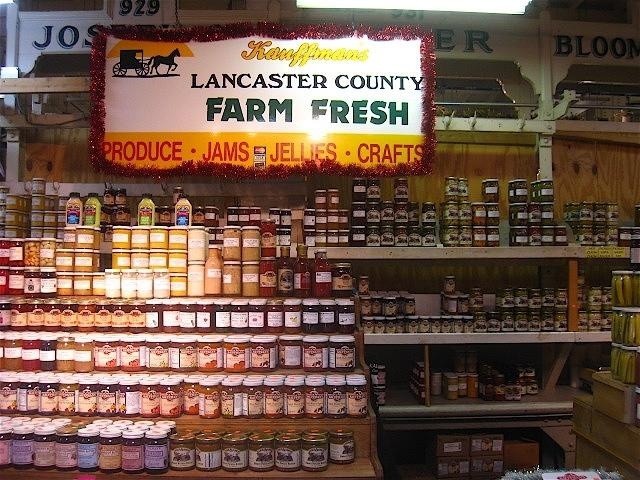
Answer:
[0,1,640,429]
[571,396,640,479]
[98,241,632,471]
[0,294,384,479]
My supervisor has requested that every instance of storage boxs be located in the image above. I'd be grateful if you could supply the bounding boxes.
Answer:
[431,432,470,457]
[472,456,504,480]
[432,457,470,480]
[503,437,539,465]
[542,426,576,469]
[468,434,504,455]
[591,371,635,424]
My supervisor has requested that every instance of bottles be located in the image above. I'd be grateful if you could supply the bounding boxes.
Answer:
[312,249,332,298]
[66,192,83,227]
[204,244,222,295]
[218,247,224,294]
[174,193,192,225]
[277,246,294,296]
[84,193,101,227]
[137,193,155,225]
[294,245,312,299]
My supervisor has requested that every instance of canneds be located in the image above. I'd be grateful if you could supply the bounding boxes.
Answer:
[561,199,617,247]
[508,179,529,247]
[260,208,292,295]
[112,226,187,273]
[473,178,500,247]
[479,360,538,401]
[231,299,284,334]
[430,351,479,400]
[187,226,209,296]
[284,298,354,334]
[250,334,355,372]
[146,297,231,334]
[616,204,640,248]
[23,268,57,299]
[441,316,474,333]
[440,291,469,315]
[170,273,187,297]
[61,295,145,334]
[0,296,61,331]
[31,178,70,239]
[222,205,261,295]
[439,175,473,247]
[528,179,568,246]
[329,428,355,465]
[0,238,24,295]
[577,264,585,285]
[101,188,131,242]
[405,316,441,333]
[184,375,286,419]
[169,426,251,472]
[249,428,329,472]
[172,334,253,372]
[578,286,613,331]
[74,333,171,372]
[349,176,394,247]
[0,185,31,238]
[77,419,176,475]
[362,316,405,333]
[73,374,188,418]
[0,416,92,471]
[56,226,105,296]
[193,206,218,227]
[208,226,223,246]
[0,332,74,371]
[284,374,367,418]
[155,186,183,225]
[358,274,370,296]
[0,372,78,416]
[106,268,170,299]
[378,290,415,316]
[469,287,487,333]
[444,275,455,294]
[304,188,351,247]
[408,359,425,405]
[611,271,640,385]
[487,288,528,331]
[329,261,352,298]
[393,178,436,248]
[528,285,567,332]
[360,291,382,316]
